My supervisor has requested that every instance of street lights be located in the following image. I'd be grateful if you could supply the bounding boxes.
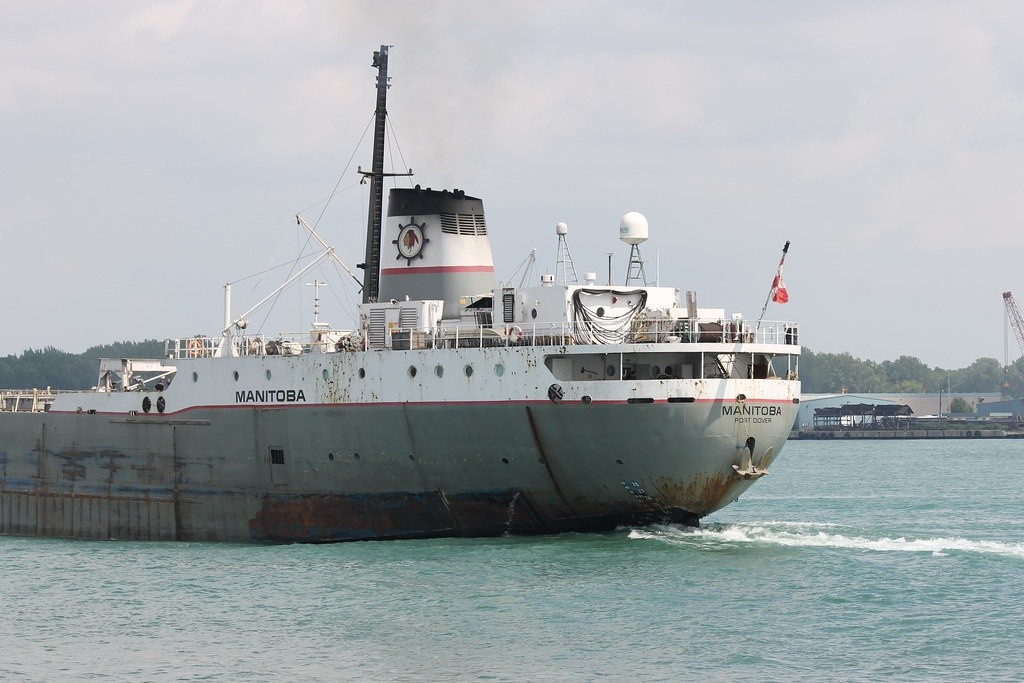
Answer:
[939,386,943,424]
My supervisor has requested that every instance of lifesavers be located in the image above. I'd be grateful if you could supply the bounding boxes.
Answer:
[975,431,981,437]
[508,326,523,343]
[844,431,850,438]
[967,431,973,437]
[188,340,202,355]
[799,431,835,439]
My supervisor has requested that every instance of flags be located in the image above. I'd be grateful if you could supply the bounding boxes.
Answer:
[771,260,789,304]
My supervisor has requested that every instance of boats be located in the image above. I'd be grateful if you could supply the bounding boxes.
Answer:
[0,44,801,544]
